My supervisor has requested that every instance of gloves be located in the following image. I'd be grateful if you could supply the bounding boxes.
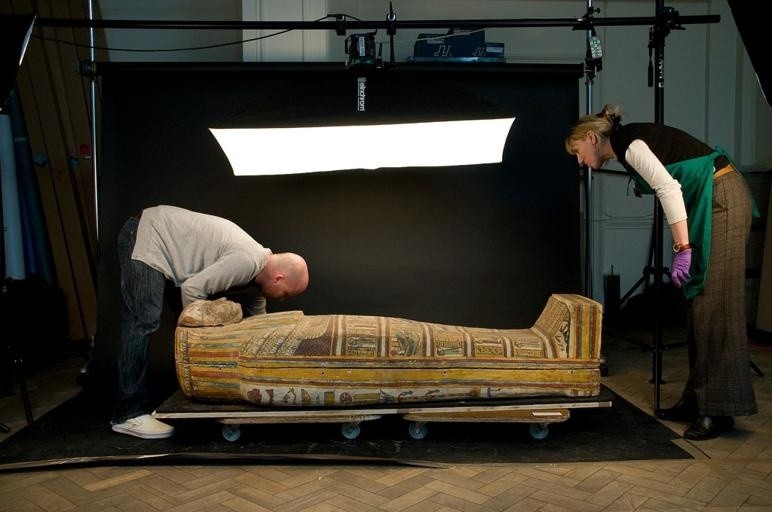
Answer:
[669,250,693,287]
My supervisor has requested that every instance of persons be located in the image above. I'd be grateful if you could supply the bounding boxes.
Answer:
[110,204,310,440]
[566,102,758,441]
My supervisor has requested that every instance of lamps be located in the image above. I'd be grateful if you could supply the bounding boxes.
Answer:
[208,34,518,177]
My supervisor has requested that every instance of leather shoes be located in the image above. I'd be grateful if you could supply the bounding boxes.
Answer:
[685,412,736,439]
[653,395,700,421]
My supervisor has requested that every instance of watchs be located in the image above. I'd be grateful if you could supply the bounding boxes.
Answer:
[674,242,692,254]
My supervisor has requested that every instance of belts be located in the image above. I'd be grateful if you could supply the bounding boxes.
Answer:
[710,162,735,180]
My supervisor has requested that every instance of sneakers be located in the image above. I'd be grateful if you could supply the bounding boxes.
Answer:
[109,415,178,440]
